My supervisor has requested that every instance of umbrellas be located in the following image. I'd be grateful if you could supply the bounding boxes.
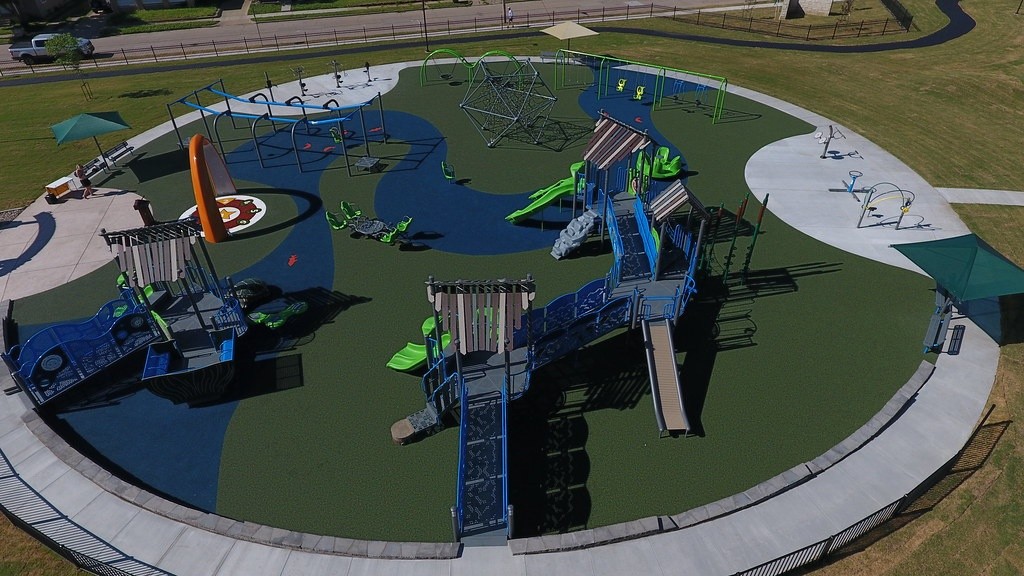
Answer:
[540,21,600,64]
[891,234,1024,317]
[50,111,132,170]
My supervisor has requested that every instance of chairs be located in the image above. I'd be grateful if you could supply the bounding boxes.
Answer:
[442,162,455,183]
[372,229,399,243]
[616,78,627,91]
[326,211,348,229]
[329,127,342,143]
[396,216,413,234]
[341,201,362,219]
[633,86,645,99]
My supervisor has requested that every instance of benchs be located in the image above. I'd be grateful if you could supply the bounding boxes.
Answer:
[540,52,563,64]
[923,312,951,353]
[935,282,952,313]
[104,141,134,167]
[74,158,106,179]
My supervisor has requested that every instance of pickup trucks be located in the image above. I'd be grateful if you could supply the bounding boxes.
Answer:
[7,34,96,58]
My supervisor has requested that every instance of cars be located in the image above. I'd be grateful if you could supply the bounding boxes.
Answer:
[90,0,110,14]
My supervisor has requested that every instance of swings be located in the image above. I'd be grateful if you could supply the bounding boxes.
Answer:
[584,55,596,87]
[616,59,629,92]
[633,63,648,101]
[694,73,711,105]
[672,71,688,102]
[567,53,580,84]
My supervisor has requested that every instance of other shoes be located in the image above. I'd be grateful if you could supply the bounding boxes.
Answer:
[83,195,87,199]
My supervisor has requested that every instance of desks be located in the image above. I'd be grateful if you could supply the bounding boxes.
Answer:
[355,157,379,173]
[45,177,78,200]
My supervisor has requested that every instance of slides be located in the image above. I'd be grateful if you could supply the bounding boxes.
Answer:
[639,318,692,432]
[505,160,585,224]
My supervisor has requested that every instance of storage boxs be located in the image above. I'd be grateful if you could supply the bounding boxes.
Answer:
[94,161,105,169]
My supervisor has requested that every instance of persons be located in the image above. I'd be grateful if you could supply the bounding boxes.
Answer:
[696,100,701,107]
[76,163,96,199]
[632,176,643,196]
[507,7,514,28]
